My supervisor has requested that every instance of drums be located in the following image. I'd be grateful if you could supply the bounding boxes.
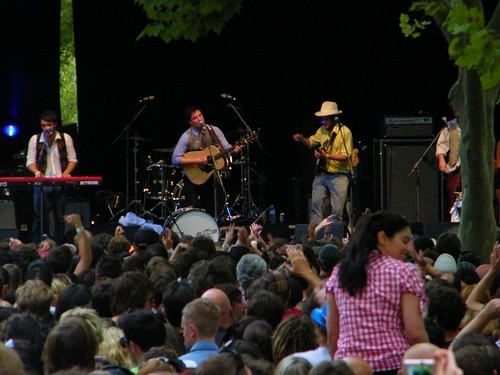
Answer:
[139,147,186,202]
[162,206,220,243]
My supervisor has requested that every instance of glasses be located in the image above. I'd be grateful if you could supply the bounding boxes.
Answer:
[119,336,130,348]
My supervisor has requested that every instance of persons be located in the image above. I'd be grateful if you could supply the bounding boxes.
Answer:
[326,210,430,375]
[26,108,79,245]
[39,316,98,375]
[0,209,500,375]
[177,296,222,369]
[291,100,354,243]
[171,107,244,208]
[436,105,462,224]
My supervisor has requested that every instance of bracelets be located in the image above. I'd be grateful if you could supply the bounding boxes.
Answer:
[76,225,86,234]
[34,170,42,176]
[326,154,330,159]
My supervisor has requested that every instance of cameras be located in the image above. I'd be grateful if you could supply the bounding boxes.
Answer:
[402,358,435,375]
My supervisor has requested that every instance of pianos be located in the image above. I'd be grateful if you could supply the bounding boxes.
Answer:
[0,175,103,246]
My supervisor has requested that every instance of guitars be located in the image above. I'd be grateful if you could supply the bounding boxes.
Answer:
[312,147,327,176]
[180,130,261,186]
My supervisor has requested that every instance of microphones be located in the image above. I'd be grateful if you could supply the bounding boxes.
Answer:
[221,93,236,101]
[139,96,155,102]
[199,121,205,130]
[441,117,447,124]
[334,117,343,126]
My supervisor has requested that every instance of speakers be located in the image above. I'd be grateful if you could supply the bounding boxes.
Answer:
[0,200,18,228]
[410,222,459,235]
[295,224,351,241]
[386,146,439,224]
[0,228,30,244]
[60,226,115,245]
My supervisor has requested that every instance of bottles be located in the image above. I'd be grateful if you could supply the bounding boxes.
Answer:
[269,205,275,225]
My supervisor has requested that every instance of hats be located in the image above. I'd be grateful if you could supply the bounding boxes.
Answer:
[309,303,327,330]
[133,226,159,249]
[262,267,290,296]
[433,253,457,273]
[315,101,343,116]
